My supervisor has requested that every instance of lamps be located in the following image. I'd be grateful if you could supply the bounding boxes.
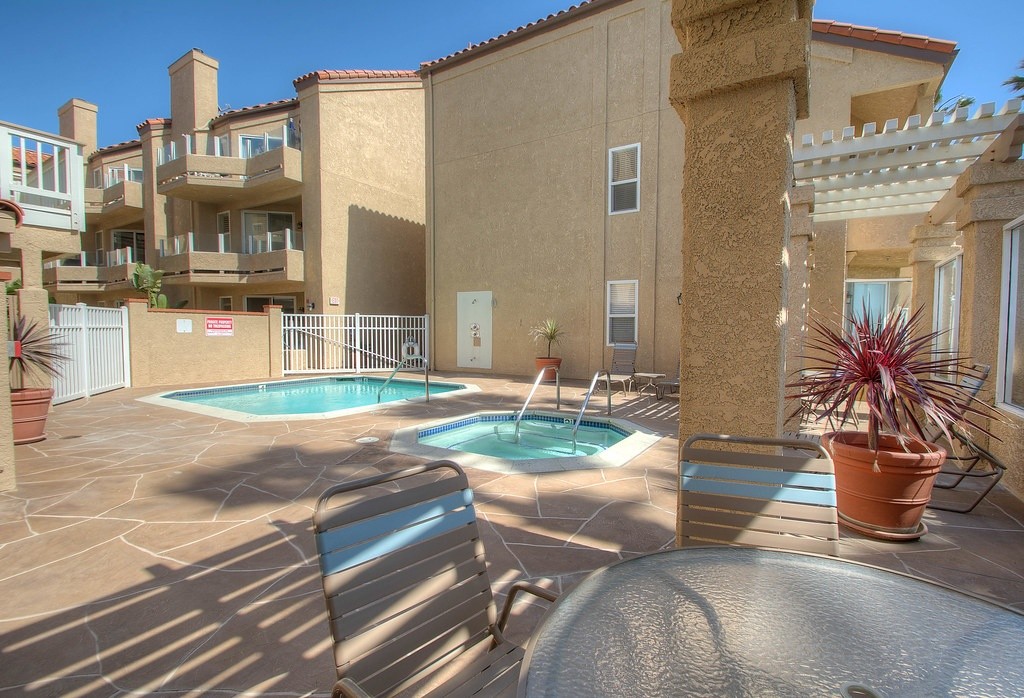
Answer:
[307,302,315,312]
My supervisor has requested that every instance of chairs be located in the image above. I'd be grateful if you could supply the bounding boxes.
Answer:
[655,359,680,400]
[916,361,1006,513]
[673,432,839,558]
[314,457,557,698]
[592,338,637,396]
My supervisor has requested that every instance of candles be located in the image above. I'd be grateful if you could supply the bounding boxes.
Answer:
[529,318,567,381]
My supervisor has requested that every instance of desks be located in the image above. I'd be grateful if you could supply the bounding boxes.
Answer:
[511,544,1024,698]
[634,372,666,397]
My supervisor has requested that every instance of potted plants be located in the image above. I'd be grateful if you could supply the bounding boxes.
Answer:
[782,296,1020,532]
[10,314,76,438]
